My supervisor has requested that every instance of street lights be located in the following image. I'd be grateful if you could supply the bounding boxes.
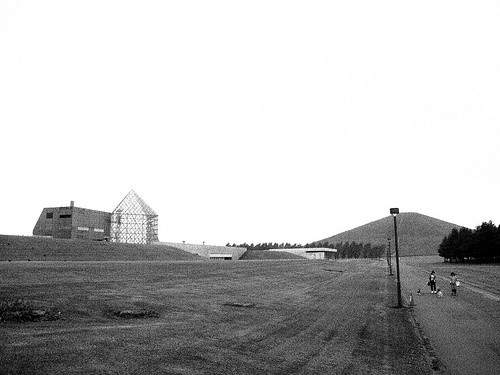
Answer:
[387,237,393,276]
[389,208,406,309]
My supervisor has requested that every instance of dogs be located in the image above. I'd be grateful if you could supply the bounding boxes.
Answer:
[436,289,443,298]
[417,288,424,296]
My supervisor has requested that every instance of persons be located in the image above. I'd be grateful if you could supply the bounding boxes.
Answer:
[449,272,457,296]
[428,271,437,294]
[437,289,443,299]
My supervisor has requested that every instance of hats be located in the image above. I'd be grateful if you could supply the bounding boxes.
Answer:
[451,272,457,276]
[431,271,435,275]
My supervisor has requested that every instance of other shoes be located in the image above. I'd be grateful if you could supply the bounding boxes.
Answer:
[431,291,437,293]
